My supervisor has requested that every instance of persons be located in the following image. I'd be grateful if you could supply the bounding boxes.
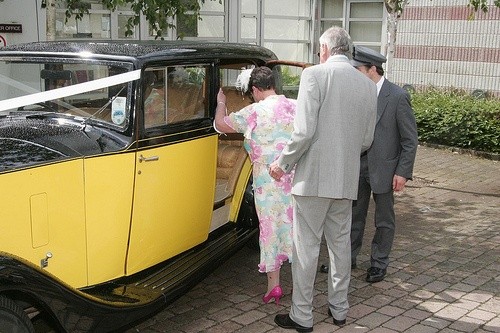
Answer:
[213,65,300,307]
[320,45,419,284]
[142,67,204,128]
[268,26,377,333]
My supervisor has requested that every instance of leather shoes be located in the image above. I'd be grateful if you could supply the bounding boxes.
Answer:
[366,266,386,282]
[320,262,357,274]
[327,307,346,325]
[274,313,313,333]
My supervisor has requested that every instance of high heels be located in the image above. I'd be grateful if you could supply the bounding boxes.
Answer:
[263,285,282,306]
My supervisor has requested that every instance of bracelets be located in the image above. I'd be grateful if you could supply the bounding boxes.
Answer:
[218,101,225,105]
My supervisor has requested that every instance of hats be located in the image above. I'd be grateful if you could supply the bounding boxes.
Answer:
[350,45,387,67]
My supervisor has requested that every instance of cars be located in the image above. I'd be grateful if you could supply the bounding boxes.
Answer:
[0,39,320,333]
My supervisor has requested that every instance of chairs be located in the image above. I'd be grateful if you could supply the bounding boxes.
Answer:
[143,86,257,179]
